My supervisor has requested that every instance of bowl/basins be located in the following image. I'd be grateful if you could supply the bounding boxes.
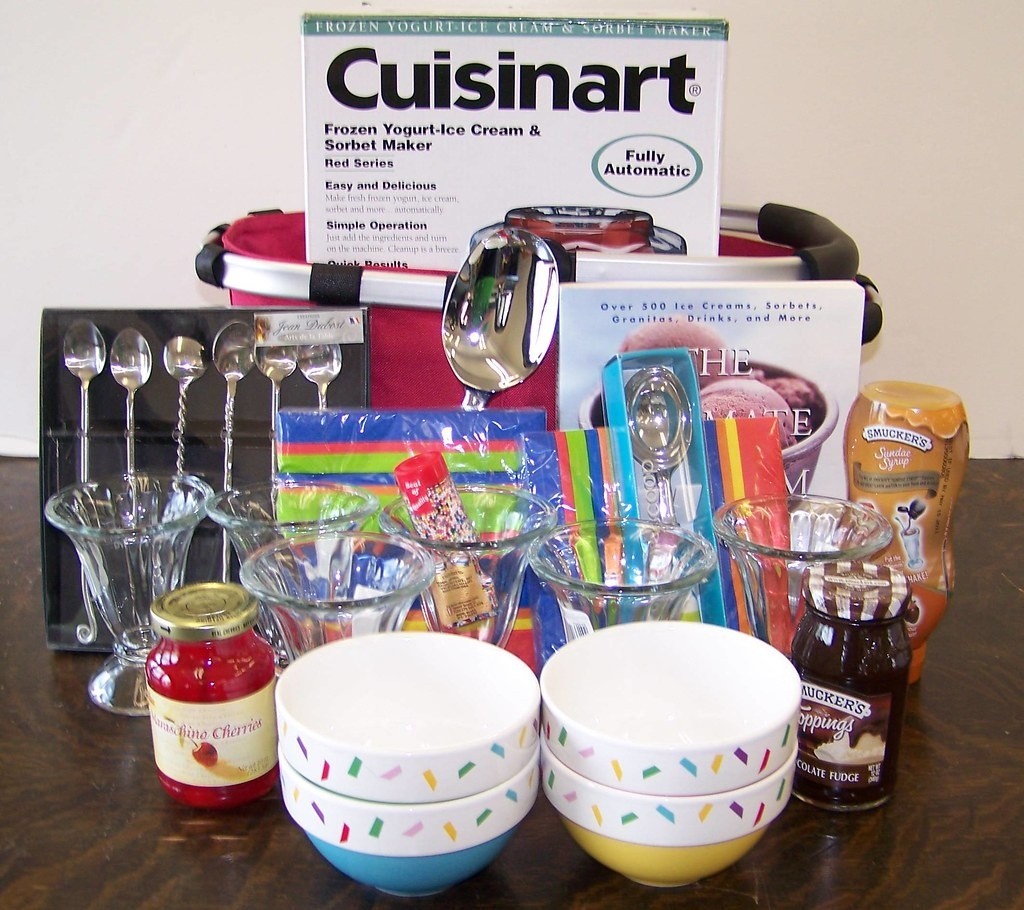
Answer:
[579,354,840,510]
[271,619,806,898]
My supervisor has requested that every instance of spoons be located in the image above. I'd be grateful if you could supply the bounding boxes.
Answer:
[212,319,256,584]
[256,345,299,525]
[440,228,558,413]
[301,342,344,413]
[110,327,161,473]
[627,364,694,525]
[60,317,107,646]
[162,335,207,473]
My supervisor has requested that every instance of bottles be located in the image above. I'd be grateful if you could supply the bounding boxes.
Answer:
[840,380,971,684]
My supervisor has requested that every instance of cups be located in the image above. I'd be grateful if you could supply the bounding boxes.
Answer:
[526,518,716,650]
[202,480,379,676]
[373,484,557,652]
[237,530,435,670]
[42,475,214,718]
[714,495,894,655]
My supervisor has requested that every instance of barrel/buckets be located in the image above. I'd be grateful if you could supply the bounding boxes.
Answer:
[193,199,878,408]
